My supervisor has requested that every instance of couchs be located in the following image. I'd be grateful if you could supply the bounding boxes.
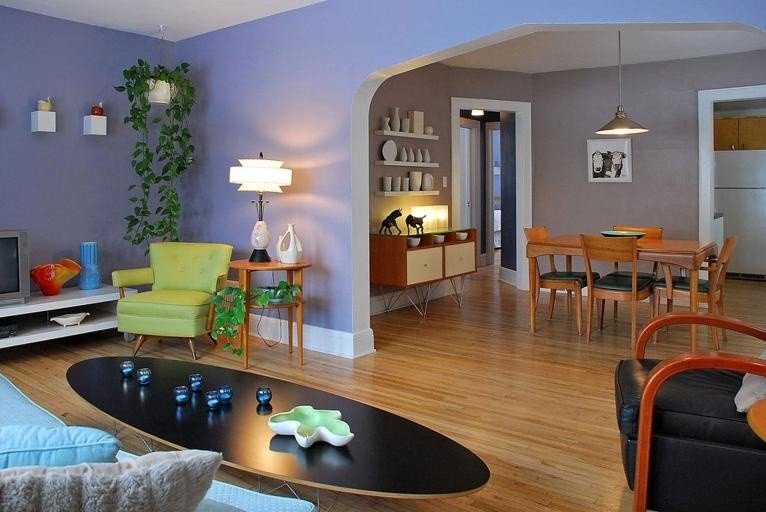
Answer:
[112,242,233,360]
[1,372,316,512]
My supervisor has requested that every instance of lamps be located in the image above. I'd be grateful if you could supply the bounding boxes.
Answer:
[595,31,649,135]
[229,152,292,264]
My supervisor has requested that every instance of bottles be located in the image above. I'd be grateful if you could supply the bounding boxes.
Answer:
[400,146,431,162]
[116,359,277,408]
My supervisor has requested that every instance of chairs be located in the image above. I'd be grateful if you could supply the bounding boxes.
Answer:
[613,312,764,512]
[524,224,741,355]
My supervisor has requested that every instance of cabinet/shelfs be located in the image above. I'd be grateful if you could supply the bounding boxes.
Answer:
[714,116,765,151]
[370,228,476,319]
[374,130,439,196]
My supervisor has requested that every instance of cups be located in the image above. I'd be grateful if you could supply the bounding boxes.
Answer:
[381,176,410,192]
[422,173,434,191]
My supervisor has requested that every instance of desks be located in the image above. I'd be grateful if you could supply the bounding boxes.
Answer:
[1,282,138,349]
[230,260,312,369]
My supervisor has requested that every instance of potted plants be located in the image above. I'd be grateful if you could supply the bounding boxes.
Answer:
[207,280,301,358]
[113,58,197,256]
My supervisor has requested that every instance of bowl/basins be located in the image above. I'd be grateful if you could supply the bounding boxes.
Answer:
[600,231,647,240]
[432,235,445,244]
[456,232,469,241]
[407,237,421,248]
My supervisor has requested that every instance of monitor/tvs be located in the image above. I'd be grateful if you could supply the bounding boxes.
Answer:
[0,229,31,305]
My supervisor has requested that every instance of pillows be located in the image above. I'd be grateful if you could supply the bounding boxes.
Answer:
[0,449,222,512]
[733,351,766,413]
[1,425,120,469]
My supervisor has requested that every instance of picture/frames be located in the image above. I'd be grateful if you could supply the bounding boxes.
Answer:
[586,138,633,182]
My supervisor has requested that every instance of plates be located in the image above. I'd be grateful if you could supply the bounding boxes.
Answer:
[382,139,398,161]
